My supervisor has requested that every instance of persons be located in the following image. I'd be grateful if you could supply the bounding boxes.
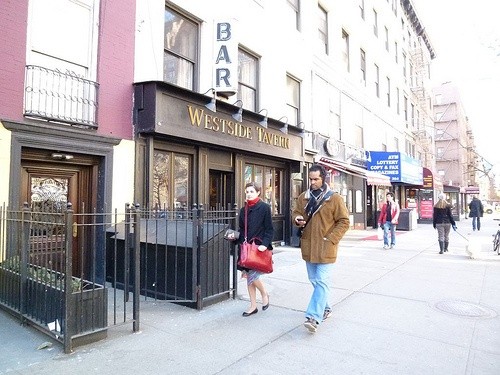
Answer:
[237,182,273,316]
[433,193,456,253]
[291,165,350,334]
[469,195,484,231]
[378,192,399,249]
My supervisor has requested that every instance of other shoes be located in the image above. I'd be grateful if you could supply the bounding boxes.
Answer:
[262,295,269,310]
[390,245,394,250]
[242,308,258,317]
[321,309,332,322]
[304,318,318,332]
[383,244,388,250]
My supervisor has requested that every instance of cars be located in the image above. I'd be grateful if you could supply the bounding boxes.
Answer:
[483,203,494,214]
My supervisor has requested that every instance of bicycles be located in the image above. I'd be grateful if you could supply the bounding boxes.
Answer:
[491,219,500,256]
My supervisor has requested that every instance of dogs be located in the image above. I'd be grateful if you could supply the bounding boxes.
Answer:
[465,234,483,260]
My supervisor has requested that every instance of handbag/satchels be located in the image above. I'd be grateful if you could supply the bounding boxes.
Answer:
[238,237,274,274]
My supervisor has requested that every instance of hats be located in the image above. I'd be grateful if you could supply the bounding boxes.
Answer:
[439,193,446,200]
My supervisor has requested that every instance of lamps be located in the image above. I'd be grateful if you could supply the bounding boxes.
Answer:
[296,124,305,138]
[257,109,268,129]
[202,88,217,113]
[231,100,243,123]
[278,117,289,135]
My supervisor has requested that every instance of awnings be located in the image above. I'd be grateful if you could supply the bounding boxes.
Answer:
[444,184,480,194]
[368,151,423,187]
[407,168,444,204]
[318,158,392,187]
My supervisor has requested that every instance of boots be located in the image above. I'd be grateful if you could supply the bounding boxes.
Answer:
[439,241,449,254]
